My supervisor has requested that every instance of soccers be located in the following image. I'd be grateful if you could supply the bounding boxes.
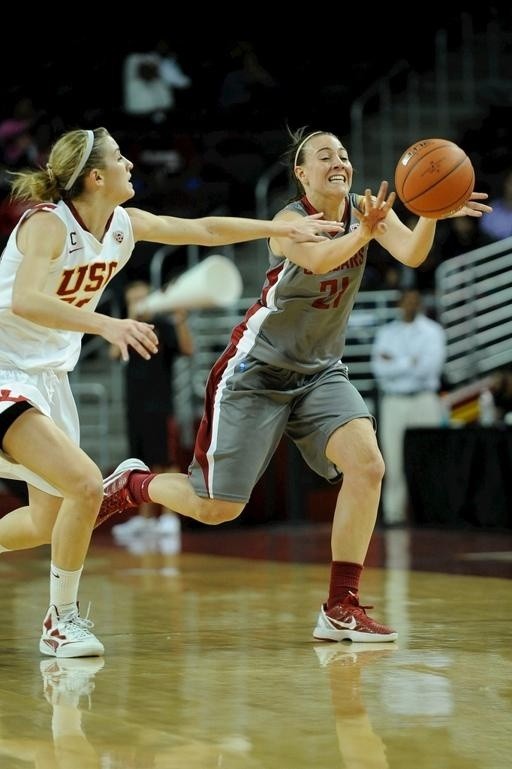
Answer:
[396,138,477,218]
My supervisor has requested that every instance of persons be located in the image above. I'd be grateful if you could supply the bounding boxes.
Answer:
[0,657,109,769]
[0,125,346,659]
[91,129,495,642]
[369,177,512,527]
[313,640,396,769]
[106,281,195,544]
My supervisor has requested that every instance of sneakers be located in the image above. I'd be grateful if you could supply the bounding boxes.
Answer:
[312,591,398,643]
[39,601,105,658]
[110,513,180,537]
[90,457,152,529]
